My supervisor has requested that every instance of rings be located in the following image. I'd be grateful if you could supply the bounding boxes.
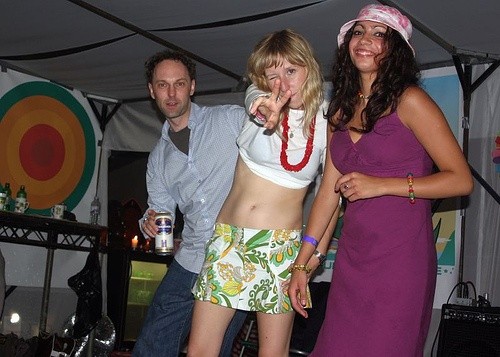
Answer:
[343,182,349,190]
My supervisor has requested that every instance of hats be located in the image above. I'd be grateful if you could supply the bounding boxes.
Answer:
[337,4,416,58]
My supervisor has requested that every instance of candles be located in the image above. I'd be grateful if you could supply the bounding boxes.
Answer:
[131,236,139,249]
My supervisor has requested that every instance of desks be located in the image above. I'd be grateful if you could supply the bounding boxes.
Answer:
[0,209,110,357]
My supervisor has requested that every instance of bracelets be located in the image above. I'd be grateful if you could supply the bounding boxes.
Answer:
[289,266,311,272]
[312,250,325,264]
[407,173,415,204]
[301,233,318,246]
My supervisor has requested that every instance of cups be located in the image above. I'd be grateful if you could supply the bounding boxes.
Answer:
[0,193,7,210]
[14,198,29,213]
[50,205,66,220]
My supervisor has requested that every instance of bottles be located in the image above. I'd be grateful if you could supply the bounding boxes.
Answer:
[17,185,26,198]
[90,198,101,225]
[2,183,11,211]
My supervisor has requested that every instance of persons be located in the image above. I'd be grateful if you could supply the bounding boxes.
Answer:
[189,27,344,357]
[130,50,244,357]
[286,4,475,357]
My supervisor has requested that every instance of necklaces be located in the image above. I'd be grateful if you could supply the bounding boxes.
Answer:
[278,103,317,170]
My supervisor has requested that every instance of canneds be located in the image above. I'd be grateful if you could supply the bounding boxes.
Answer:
[51,203,67,219]
[152,212,174,254]
